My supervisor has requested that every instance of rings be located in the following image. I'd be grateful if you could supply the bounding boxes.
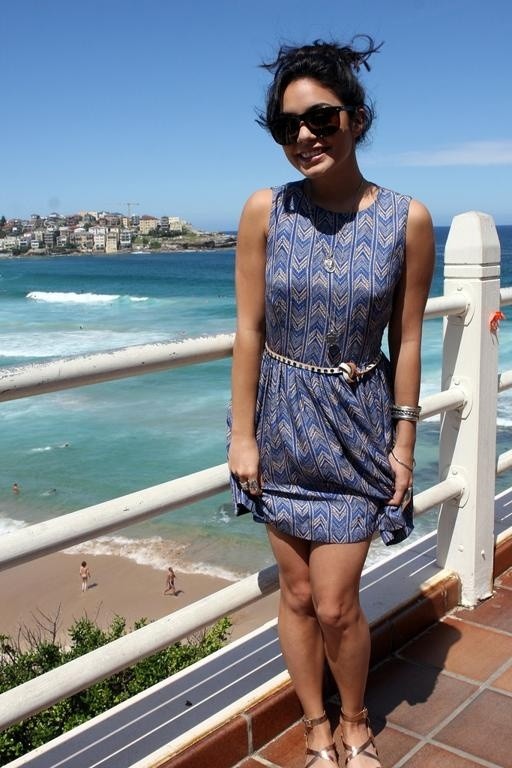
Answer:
[239,482,249,491]
[247,480,259,489]
[406,487,413,493]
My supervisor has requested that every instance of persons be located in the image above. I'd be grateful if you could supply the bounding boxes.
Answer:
[228,35,436,768]
[80,562,91,593]
[12,484,19,496]
[163,567,177,596]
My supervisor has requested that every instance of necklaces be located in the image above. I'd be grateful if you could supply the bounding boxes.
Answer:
[305,180,364,272]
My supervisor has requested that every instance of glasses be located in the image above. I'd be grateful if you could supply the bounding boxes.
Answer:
[270,106,359,145]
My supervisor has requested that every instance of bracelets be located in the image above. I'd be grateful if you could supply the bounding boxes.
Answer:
[391,405,422,422]
[391,451,416,471]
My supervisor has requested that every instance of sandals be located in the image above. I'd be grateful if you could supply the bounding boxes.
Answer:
[302,710,338,768]
[340,707,383,768]
